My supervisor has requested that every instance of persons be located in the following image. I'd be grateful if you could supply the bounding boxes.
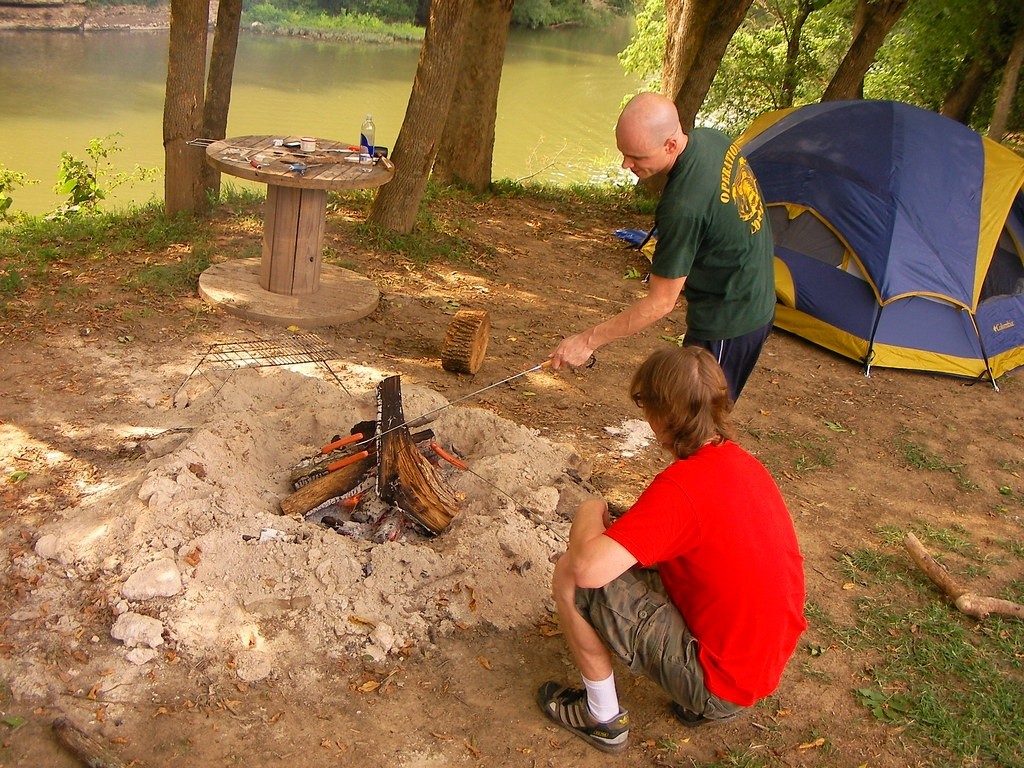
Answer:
[537,344,808,754]
[547,91,777,420]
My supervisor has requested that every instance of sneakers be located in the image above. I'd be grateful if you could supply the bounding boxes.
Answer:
[536,680,630,753]
[671,697,735,728]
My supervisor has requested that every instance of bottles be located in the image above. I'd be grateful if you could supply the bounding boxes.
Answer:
[358,115,375,172]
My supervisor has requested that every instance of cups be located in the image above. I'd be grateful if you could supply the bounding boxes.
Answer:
[300,137,317,152]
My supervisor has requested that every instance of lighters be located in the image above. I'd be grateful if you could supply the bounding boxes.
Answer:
[251,161,262,169]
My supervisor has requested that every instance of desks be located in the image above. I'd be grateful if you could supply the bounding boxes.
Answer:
[197,135,395,328]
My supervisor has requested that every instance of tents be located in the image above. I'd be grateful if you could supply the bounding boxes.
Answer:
[615,100,1024,392]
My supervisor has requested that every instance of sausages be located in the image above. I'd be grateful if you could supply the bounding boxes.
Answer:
[321,433,363,453]
[326,450,368,471]
[430,441,469,471]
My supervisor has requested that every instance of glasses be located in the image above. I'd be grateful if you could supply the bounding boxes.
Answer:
[632,391,648,409]
